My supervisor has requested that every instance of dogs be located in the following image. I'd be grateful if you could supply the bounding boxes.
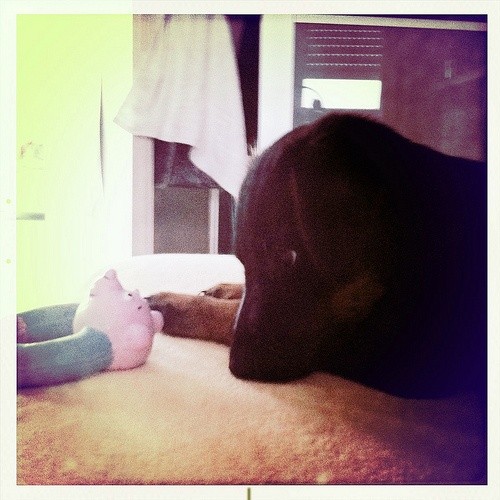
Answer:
[145,109,488,399]
